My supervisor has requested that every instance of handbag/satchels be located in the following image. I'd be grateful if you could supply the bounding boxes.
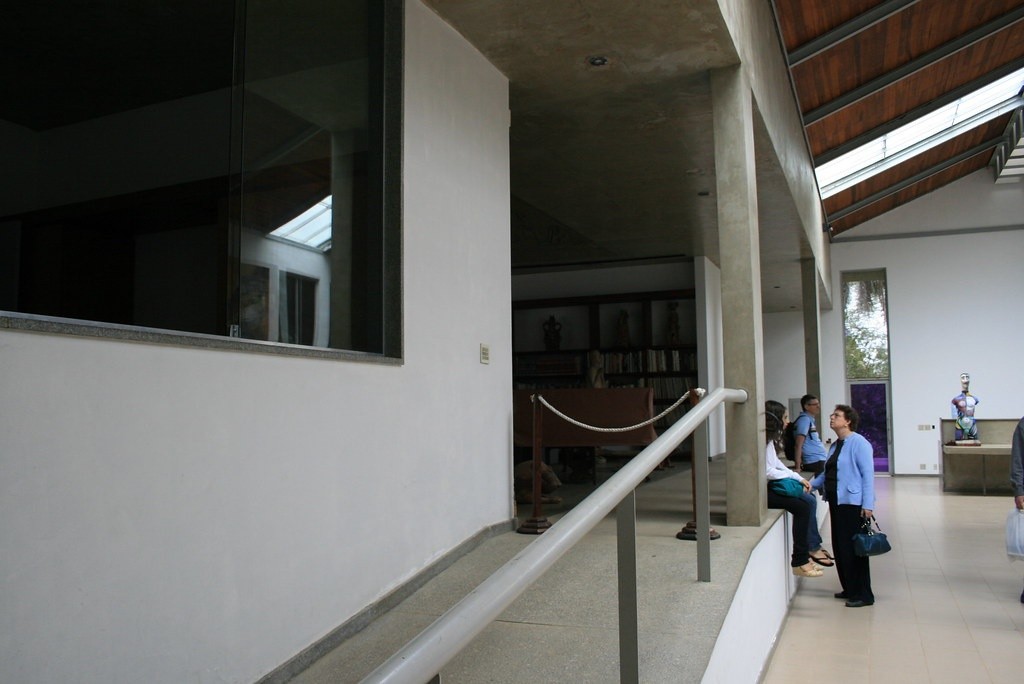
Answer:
[1006,508,1024,558]
[852,514,891,557]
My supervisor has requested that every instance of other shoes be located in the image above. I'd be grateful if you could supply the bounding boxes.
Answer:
[846,599,874,606]
[834,592,853,598]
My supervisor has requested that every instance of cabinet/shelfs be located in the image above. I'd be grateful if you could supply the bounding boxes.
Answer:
[513,344,700,463]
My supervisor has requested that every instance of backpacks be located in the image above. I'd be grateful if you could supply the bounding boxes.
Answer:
[783,415,812,460]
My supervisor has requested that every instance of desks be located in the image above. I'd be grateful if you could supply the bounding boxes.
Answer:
[944,443,1013,496]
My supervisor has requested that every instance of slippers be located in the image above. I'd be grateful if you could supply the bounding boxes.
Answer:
[820,549,835,560]
[808,552,833,566]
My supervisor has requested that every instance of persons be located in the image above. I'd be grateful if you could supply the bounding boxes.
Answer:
[1011,416,1024,604]
[952,372,979,440]
[766,394,875,607]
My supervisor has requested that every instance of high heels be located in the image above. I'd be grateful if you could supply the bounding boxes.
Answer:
[812,562,824,570]
[793,564,823,577]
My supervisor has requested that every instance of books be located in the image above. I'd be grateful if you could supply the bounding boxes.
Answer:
[515,349,698,455]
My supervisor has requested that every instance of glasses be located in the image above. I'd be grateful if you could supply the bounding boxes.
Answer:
[807,404,819,406]
